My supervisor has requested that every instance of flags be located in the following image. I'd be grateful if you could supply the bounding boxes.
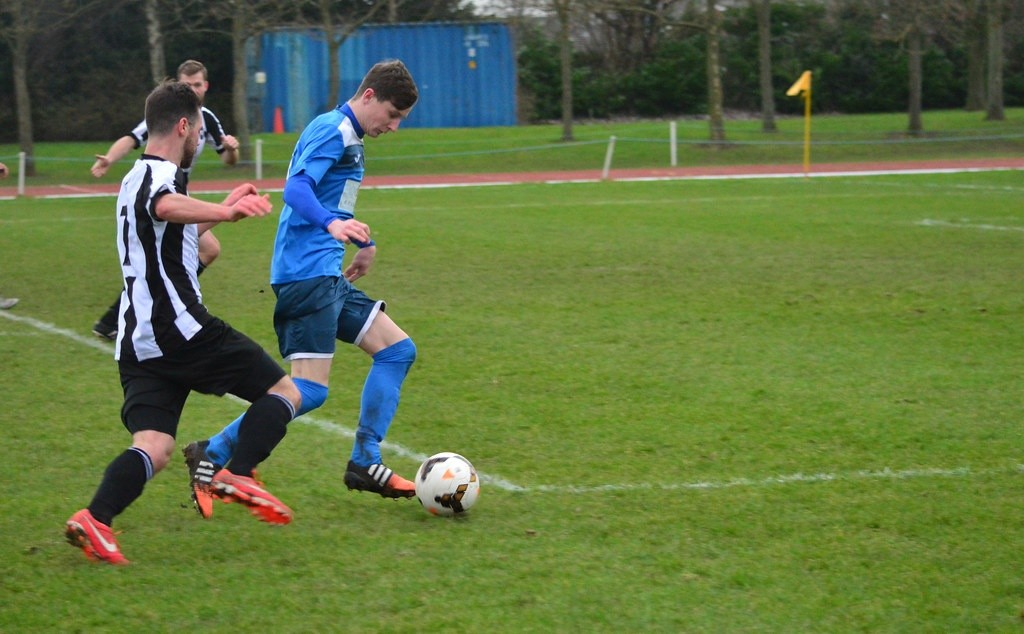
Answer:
[786,72,810,95]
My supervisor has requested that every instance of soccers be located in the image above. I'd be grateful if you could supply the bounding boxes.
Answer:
[414,450,481,516]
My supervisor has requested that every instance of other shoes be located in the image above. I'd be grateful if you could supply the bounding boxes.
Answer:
[0,296,19,309]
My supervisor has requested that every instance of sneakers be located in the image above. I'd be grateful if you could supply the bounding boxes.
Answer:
[207,470,295,526]
[182,440,219,518]
[64,508,128,564]
[344,460,416,501]
[91,322,119,340]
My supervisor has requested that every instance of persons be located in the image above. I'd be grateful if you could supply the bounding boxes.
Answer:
[71,82,301,566]
[0,162,20,309]
[90,59,239,341]
[184,60,416,518]
